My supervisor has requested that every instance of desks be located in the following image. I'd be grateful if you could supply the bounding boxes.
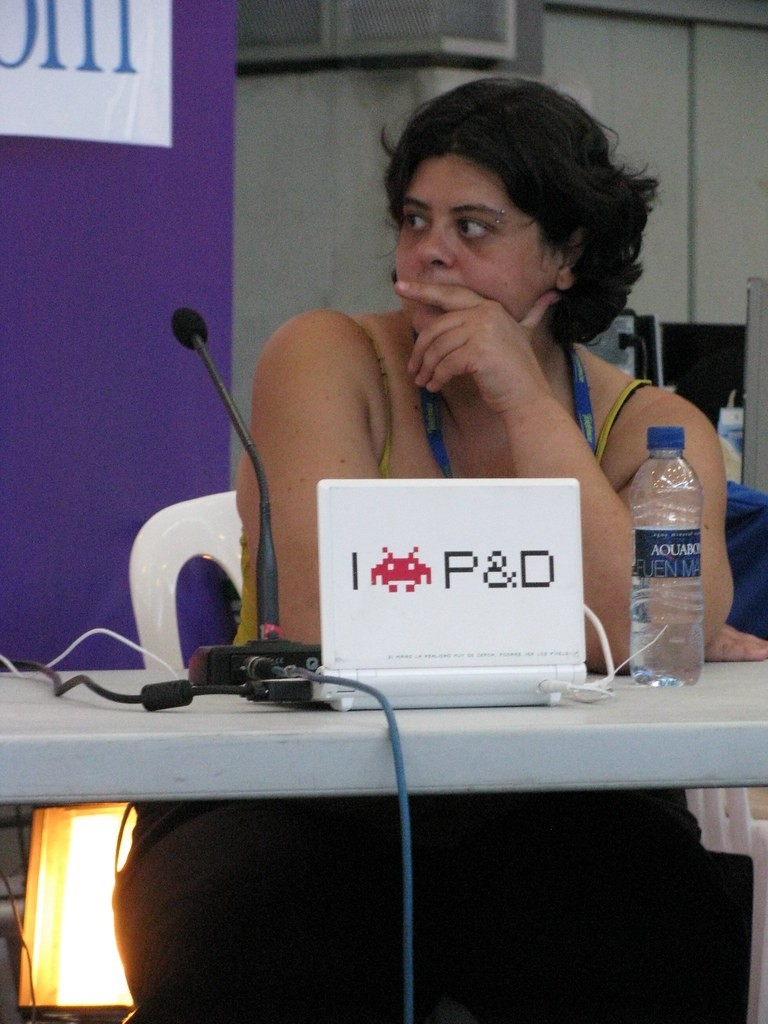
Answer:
[0,671,766,801]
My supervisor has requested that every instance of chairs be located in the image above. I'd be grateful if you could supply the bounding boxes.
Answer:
[125,487,767,1024]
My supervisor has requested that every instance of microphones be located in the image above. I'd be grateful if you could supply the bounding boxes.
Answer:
[171,307,322,688]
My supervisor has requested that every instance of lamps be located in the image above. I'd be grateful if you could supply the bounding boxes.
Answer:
[19,801,141,1024]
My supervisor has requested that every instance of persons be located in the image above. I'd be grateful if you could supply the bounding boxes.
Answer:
[112,73,753,1024]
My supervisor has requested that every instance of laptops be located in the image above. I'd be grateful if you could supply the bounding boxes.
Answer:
[245,478,588,712]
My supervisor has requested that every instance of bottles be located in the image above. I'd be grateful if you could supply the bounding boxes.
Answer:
[630,425,706,687]
